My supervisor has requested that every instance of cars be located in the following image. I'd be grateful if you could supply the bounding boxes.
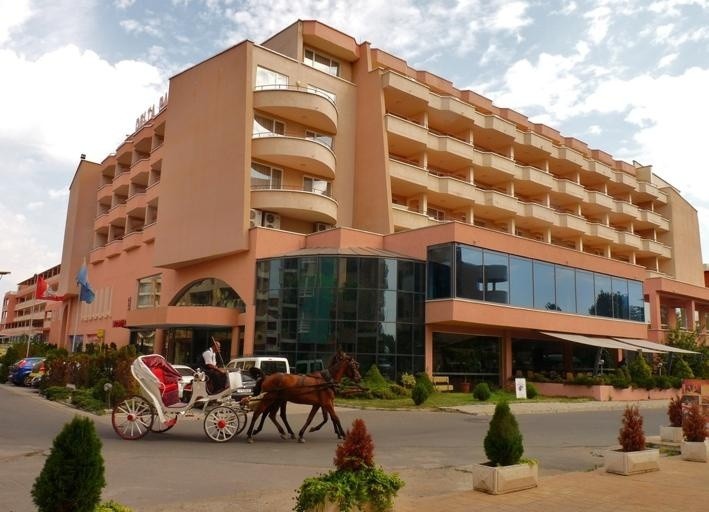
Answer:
[377,357,393,374]
[24,358,53,387]
[170,364,198,399]
[226,370,259,398]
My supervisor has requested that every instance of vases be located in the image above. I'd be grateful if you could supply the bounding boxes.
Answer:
[460,382,471,393]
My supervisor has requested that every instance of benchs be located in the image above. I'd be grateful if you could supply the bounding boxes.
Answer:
[148,366,179,407]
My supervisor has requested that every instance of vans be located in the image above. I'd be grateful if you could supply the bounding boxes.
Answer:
[226,356,291,380]
[295,358,324,377]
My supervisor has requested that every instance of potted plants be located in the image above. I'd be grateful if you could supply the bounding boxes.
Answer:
[660,395,690,442]
[474,403,541,494]
[602,403,659,475]
[681,405,709,463]
[292,415,404,511]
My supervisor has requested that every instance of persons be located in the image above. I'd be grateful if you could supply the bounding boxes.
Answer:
[202,342,227,394]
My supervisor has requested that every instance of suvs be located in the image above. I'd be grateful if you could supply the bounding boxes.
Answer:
[7,356,46,385]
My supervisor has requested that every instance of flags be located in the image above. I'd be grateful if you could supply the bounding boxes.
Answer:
[36,276,64,301]
[75,265,95,304]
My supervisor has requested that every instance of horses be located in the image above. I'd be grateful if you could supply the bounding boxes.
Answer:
[252,344,359,440]
[246,355,363,446]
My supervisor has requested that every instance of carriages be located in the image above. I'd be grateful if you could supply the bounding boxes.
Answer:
[110,350,364,446]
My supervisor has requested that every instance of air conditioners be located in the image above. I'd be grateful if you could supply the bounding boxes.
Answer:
[248,207,263,228]
[263,209,282,231]
[315,221,333,233]
